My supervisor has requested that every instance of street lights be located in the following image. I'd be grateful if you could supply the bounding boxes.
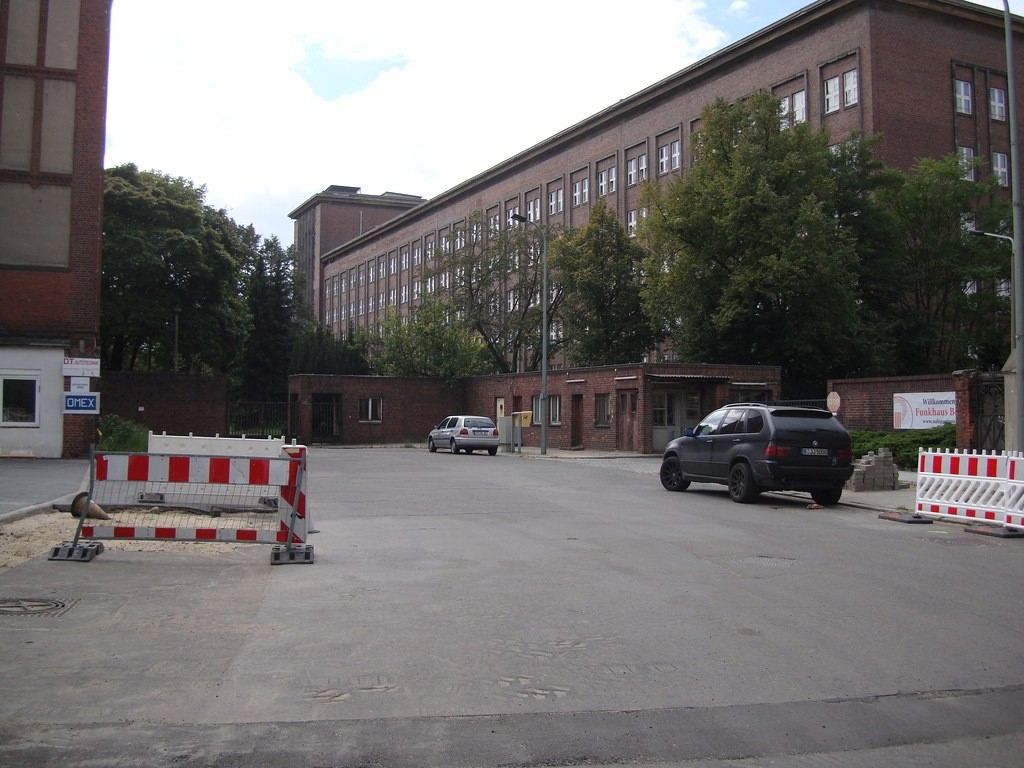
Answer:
[967,229,1016,353]
[510,213,547,455]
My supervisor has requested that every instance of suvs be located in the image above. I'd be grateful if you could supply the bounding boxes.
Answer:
[659,402,855,506]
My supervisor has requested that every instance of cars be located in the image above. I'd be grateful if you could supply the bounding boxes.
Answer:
[428,415,501,456]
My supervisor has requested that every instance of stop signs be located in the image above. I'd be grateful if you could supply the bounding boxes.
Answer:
[826,392,840,412]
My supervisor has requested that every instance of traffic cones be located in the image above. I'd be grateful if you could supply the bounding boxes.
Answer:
[70,491,111,520]
